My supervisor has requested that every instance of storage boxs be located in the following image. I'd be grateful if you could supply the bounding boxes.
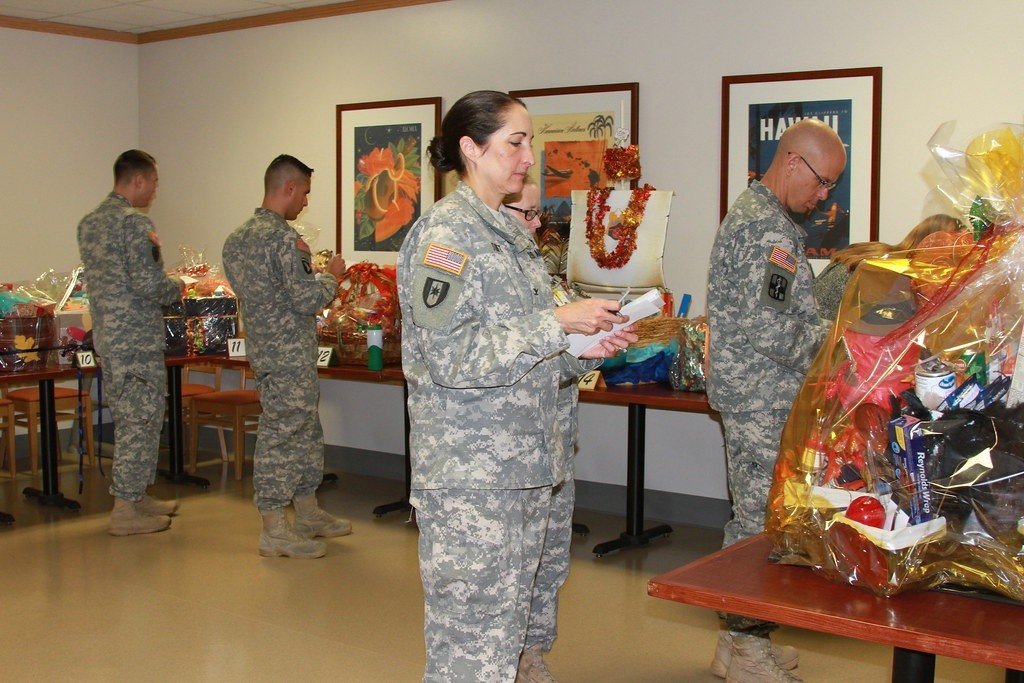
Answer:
[50,310,91,365]
[889,414,930,524]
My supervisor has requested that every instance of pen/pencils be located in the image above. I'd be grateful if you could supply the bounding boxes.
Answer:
[577,292,624,318]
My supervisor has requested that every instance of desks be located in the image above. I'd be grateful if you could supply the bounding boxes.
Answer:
[209,355,1024,683]
[0,353,222,526]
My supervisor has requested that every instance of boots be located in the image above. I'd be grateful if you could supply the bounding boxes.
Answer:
[258,507,327,558]
[134,494,179,515]
[725,633,803,683]
[515,644,558,683]
[293,494,352,539]
[108,496,171,535]
[711,618,799,679]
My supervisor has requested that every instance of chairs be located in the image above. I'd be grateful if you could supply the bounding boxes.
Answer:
[0,362,262,484]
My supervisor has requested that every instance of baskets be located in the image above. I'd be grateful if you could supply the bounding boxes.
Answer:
[629,317,688,348]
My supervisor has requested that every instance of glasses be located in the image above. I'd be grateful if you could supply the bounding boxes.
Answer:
[504,205,542,221]
[788,152,837,191]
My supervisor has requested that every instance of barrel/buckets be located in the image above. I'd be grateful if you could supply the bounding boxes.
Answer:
[162,298,238,357]
[0,316,56,374]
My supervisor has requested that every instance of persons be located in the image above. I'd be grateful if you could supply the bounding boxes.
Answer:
[397,90,638,682]
[77,149,185,536]
[814,214,970,320]
[702,115,847,682]
[222,154,352,557]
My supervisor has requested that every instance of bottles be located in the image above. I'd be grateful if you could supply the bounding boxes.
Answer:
[367,325,384,371]
[913,348,987,411]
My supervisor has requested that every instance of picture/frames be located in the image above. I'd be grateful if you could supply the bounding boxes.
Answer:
[508,82,639,284]
[336,96,442,271]
[719,66,883,282]
